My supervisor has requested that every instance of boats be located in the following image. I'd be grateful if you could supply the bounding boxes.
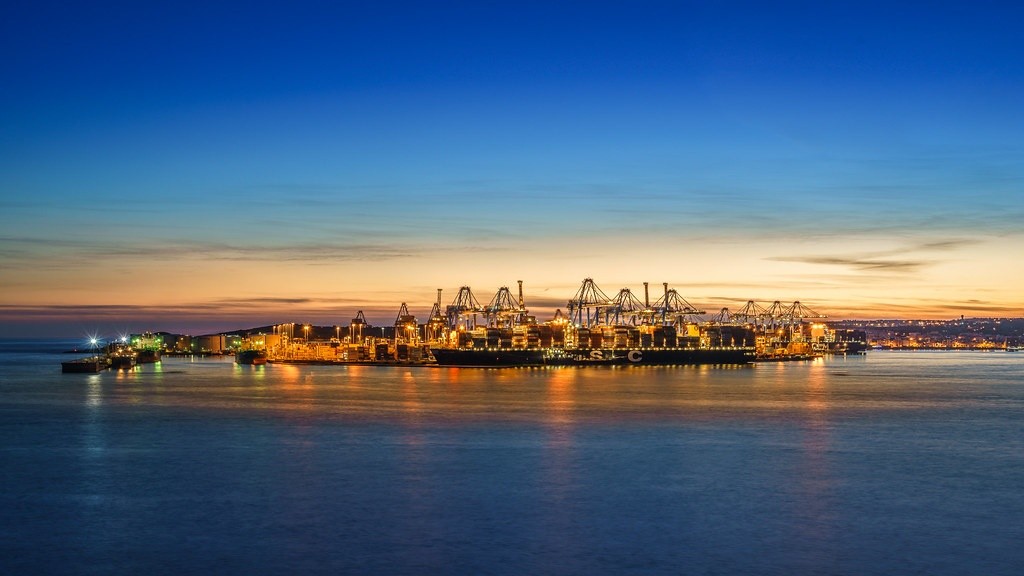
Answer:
[234,332,268,366]
[427,278,758,365]
[60,330,161,373]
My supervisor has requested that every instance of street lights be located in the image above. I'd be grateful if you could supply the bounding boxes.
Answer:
[352,324,356,343]
[349,327,351,337]
[304,326,309,343]
[336,327,340,339]
[273,323,295,338]
[382,327,384,338]
[358,324,363,342]
